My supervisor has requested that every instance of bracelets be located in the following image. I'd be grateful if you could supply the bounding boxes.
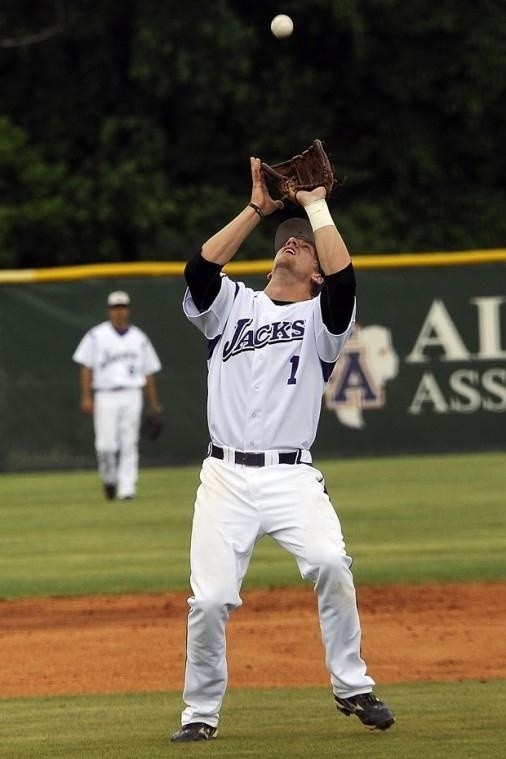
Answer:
[247,199,265,224]
[303,196,336,234]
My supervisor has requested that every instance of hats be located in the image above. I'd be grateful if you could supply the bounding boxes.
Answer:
[273,217,316,256]
[107,290,131,307]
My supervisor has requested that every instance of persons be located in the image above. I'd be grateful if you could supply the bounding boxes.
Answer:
[72,289,164,501]
[171,152,395,742]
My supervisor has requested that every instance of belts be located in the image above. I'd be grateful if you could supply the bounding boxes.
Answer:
[208,444,312,466]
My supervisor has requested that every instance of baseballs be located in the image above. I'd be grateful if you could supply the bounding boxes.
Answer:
[271,14,294,38]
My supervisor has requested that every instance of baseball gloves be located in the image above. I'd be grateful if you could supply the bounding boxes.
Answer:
[141,409,166,440]
[260,138,334,207]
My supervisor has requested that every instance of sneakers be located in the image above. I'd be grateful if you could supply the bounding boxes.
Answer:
[171,722,219,742]
[334,692,395,731]
[104,483,116,499]
